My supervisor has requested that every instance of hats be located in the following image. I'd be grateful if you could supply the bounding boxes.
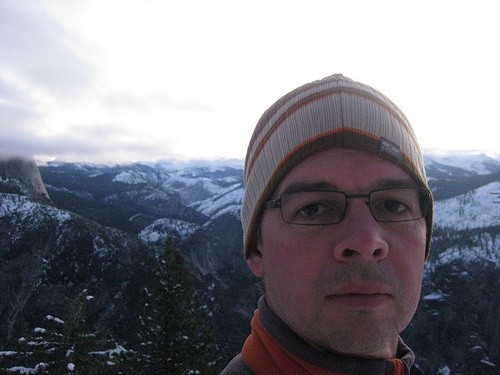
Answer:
[240,73,434,262]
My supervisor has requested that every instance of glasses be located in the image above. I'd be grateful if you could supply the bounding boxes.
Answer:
[262,187,430,226]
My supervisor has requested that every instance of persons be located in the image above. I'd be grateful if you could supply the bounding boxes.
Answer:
[218,73,434,375]
